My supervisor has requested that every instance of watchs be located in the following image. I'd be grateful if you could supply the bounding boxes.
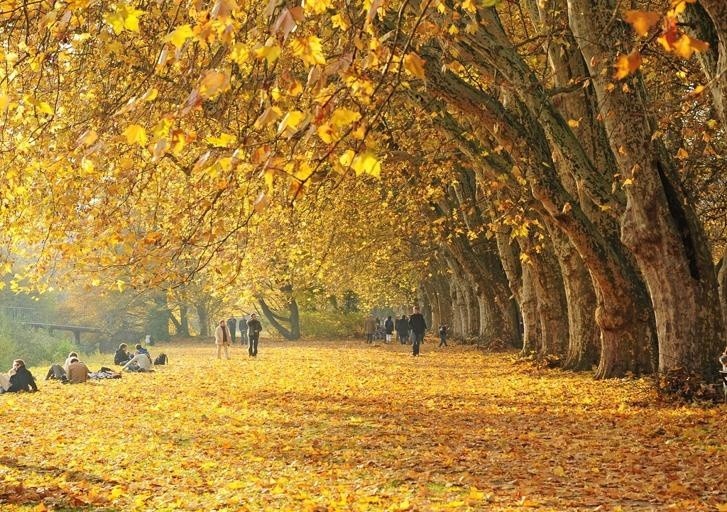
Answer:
[364,312,427,346]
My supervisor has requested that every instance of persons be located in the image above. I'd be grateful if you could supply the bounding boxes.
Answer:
[238,314,249,345]
[226,313,236,343]
[114,343,134,365]
[120,350,152,373]
[0,351,92,394]
[408,306,427,357]
[436,322,449,347]
[247,314,262,356]
[214,319,232,359]
[135,344,152,363]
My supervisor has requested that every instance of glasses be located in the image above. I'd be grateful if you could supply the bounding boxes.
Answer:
[13,363,20,366]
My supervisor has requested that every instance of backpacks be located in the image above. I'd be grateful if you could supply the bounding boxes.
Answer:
[153,352,168,365]
